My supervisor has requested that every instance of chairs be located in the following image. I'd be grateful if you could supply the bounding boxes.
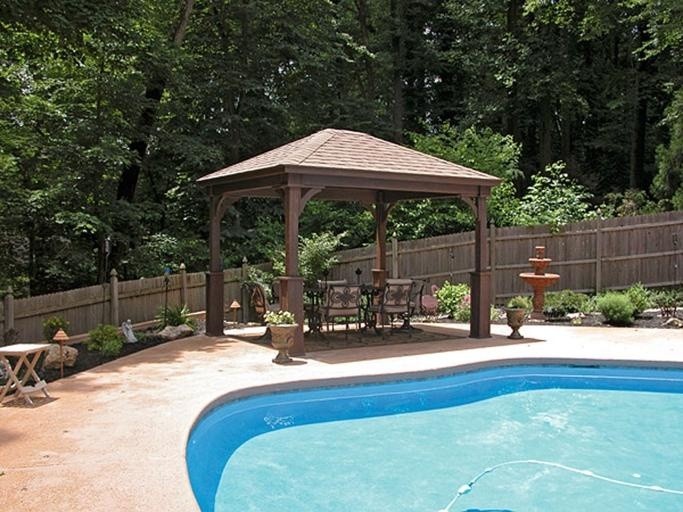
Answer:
[246,280,425,348]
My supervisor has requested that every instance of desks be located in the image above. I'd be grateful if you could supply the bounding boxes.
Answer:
[0,343,53,411]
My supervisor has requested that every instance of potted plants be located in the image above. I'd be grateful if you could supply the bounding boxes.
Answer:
[505,296,528,340]
[263,310,298,364]
